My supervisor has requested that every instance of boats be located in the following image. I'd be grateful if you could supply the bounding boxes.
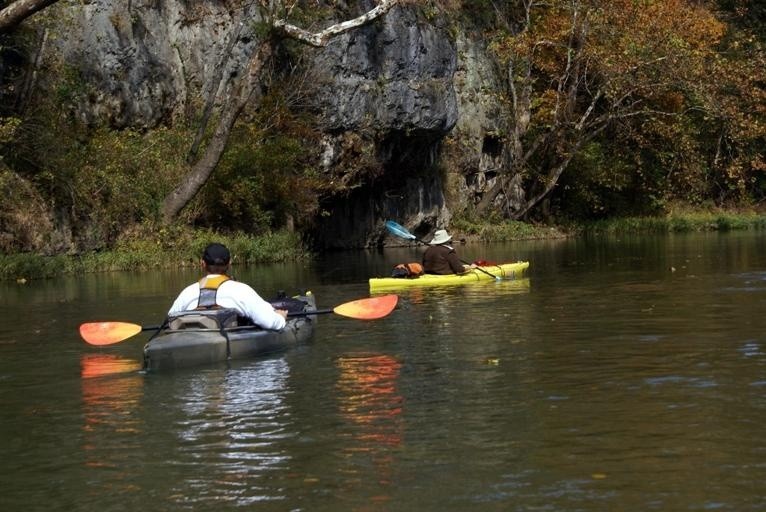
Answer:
[369,261,529,292]
[143,290,317,373]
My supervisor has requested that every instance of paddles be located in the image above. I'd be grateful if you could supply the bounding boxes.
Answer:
[80,295,398,346]
[387,221,501,279]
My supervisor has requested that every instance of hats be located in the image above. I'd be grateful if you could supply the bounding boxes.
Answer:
[426,229,454,245]
[202,242,231,266]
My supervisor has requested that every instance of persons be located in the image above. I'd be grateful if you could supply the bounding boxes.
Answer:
[167,242,289,331]
[422,229,478,276]
